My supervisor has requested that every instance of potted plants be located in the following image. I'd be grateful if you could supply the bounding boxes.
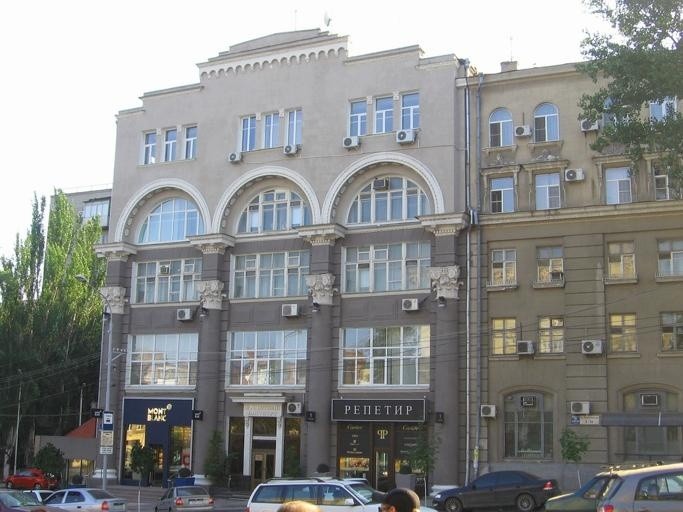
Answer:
[312,464,331,477]
[394,464,417,492]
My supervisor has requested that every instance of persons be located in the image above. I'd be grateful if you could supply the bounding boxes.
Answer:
[277,500,321,512]
[647,484,660,498]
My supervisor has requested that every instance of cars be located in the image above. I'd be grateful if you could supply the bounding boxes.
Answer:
[0,468,127,511]
[155,485,215,512]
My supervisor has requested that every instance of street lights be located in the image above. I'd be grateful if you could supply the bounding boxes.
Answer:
[75,274,114,491]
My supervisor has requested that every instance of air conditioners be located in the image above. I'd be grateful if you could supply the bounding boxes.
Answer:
[395,129,416,144]
[580,117,599,131]
[581,339,603,355]
[642,394,658,406]
[342,135,360,149]
[516,340,535,355]
[400,298,419,311]
[281,303,299,317]
[284,144,298,154]
[287,401,303,414]
[175,307,192,322]
[480,404,497,418]
[373,178,387,190]
[571,401,590,414]
[228,152,242,162]
[564,168,584,182]
[514,125,532,137]
